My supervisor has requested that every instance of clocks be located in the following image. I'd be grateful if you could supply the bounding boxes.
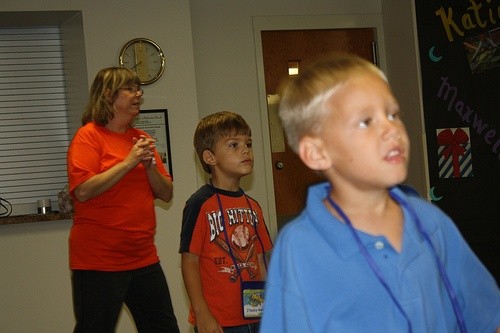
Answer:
[118,38,166,86]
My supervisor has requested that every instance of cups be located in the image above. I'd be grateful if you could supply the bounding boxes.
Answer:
[37,199,51,214]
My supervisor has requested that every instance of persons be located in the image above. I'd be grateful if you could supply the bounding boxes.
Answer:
[178,111,274,333]
[258,50,499,333]
[66,67,181,333]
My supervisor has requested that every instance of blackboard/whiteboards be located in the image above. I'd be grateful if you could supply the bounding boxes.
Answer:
[414,0,500,291]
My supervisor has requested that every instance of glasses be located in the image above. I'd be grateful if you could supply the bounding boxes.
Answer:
[119,85,137,94]
